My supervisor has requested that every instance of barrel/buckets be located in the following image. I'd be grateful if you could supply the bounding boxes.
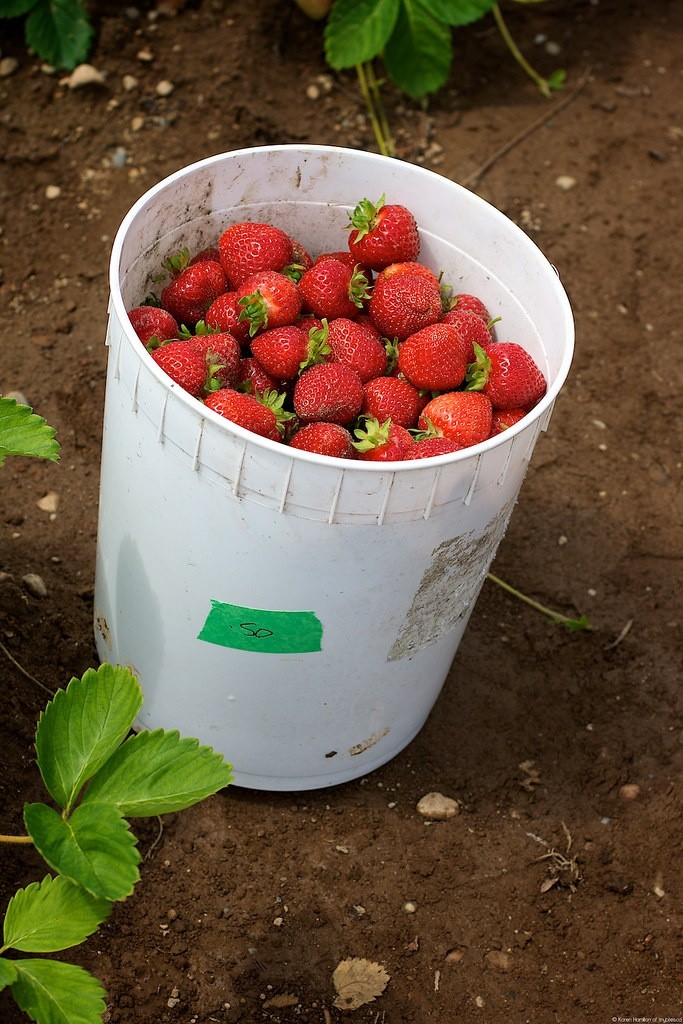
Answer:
[93,144,575,791]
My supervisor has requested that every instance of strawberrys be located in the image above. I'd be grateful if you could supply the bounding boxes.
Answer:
[296,0,332,19]
[127,192,547,463]
